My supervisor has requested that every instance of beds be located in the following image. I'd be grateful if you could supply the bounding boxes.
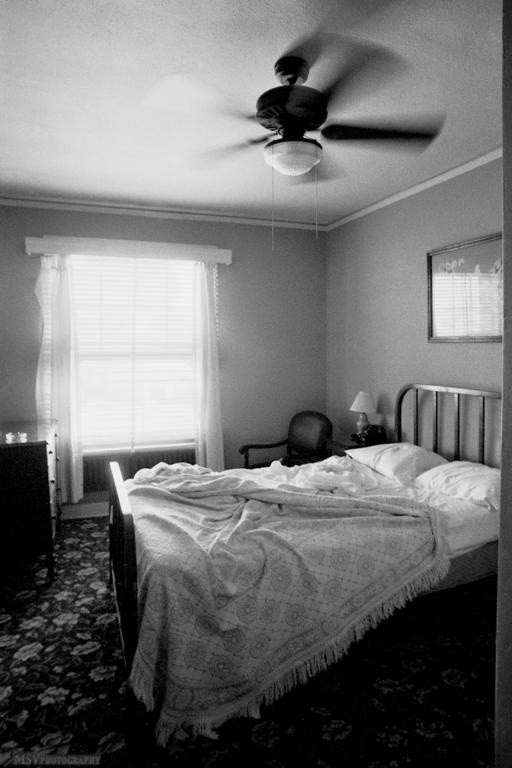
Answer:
[106,380,502,767]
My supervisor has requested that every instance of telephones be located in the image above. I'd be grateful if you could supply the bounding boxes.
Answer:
[356,424,386,447]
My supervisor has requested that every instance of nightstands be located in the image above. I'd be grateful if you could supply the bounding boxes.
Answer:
[328,435,392,457]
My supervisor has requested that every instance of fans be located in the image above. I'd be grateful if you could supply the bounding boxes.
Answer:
[202,55,437,251]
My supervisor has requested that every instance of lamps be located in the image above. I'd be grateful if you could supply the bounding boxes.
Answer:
[263,137,322,176]
[349,391,375,437]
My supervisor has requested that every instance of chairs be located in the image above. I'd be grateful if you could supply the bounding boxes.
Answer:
[238,410,333,469]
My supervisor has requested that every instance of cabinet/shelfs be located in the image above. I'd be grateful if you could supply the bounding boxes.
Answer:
[0,418,63,582]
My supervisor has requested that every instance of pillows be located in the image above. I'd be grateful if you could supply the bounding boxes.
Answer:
[343,442,501,512]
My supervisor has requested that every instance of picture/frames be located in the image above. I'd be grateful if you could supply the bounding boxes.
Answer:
[426,231,504,344]
